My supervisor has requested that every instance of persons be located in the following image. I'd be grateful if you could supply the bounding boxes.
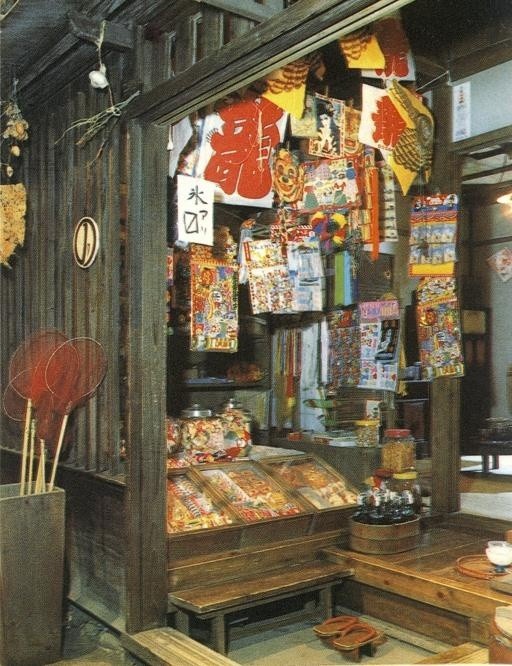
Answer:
[317,103,340,156]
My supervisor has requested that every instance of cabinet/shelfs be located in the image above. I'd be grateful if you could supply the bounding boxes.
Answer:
[175,320,270,433]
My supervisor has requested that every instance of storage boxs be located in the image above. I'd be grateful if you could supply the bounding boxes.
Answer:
[0,482,65,666]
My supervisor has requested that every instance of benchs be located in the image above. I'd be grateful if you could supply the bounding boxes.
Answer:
[167,558,355,655]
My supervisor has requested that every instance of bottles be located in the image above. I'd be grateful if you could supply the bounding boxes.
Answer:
[357,418,378,447]
[181,408,218,463]
[215,402,253,458]
[380,430,415,471]
[389,472,421,515]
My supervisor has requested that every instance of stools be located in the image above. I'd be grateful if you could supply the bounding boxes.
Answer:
[480,437,512,475]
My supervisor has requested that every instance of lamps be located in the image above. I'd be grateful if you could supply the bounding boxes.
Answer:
[495,190,512,205]
[88,20,109,89]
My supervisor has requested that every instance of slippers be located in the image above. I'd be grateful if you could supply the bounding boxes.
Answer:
[333,623,388,663]
[312,613,360,644]
